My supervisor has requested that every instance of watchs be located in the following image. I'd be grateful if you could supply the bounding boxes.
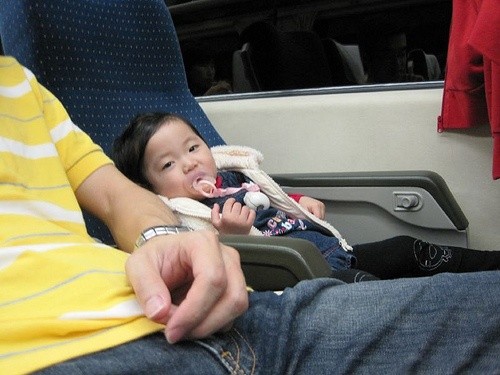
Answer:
[133,224,196,250]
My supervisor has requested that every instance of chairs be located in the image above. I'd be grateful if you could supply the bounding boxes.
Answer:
[0,1,469,291]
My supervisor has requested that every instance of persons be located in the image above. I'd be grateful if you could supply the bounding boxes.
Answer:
[0,53,500,375]
[114,111,500,283]
